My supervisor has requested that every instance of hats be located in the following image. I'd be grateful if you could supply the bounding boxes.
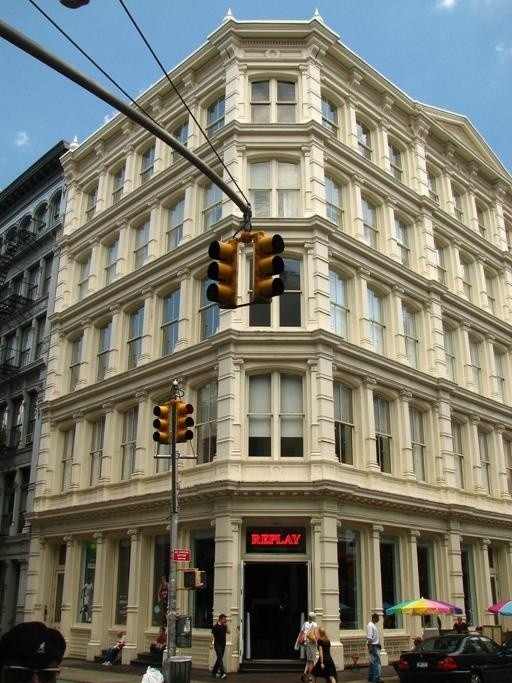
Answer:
[309,612,316,617]
[0,621,65,664]
[412,636,422,640]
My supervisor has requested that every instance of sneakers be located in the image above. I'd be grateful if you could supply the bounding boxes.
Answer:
[212,672,227,680]
[367,678,383,683]
[102,661,112,666]
[302,673,312,681]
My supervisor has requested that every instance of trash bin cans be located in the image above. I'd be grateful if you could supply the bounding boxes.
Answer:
[168,656,193,683]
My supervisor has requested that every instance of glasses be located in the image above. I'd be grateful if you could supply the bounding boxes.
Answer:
[3,666,60,682]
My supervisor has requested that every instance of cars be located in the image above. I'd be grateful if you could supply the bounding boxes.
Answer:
[398,633,511,683]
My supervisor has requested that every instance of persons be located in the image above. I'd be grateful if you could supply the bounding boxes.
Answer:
[149,627,167,663]
[298,612,318,682]
[476,627,482,635]
[437,616,441,629]
[366,613,384,683]
[102,631,126,666]
[310,627,337,683]
[157,576,168,624]
[411,637,422,652]
[209,614,231,679]
[453,616,466,634]
[80,577,93,622]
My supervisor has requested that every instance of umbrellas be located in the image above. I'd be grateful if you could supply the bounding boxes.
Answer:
[488,599,512,616]
[386,597,463,628]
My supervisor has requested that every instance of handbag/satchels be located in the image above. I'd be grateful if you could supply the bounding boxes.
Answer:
[298,632,304,644]
[306,629,316,641]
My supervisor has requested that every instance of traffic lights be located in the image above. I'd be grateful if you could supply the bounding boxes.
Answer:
[176,398,194,445]
[152,402,171,445]
[251,233,287,302]
[206,237,239,310]
